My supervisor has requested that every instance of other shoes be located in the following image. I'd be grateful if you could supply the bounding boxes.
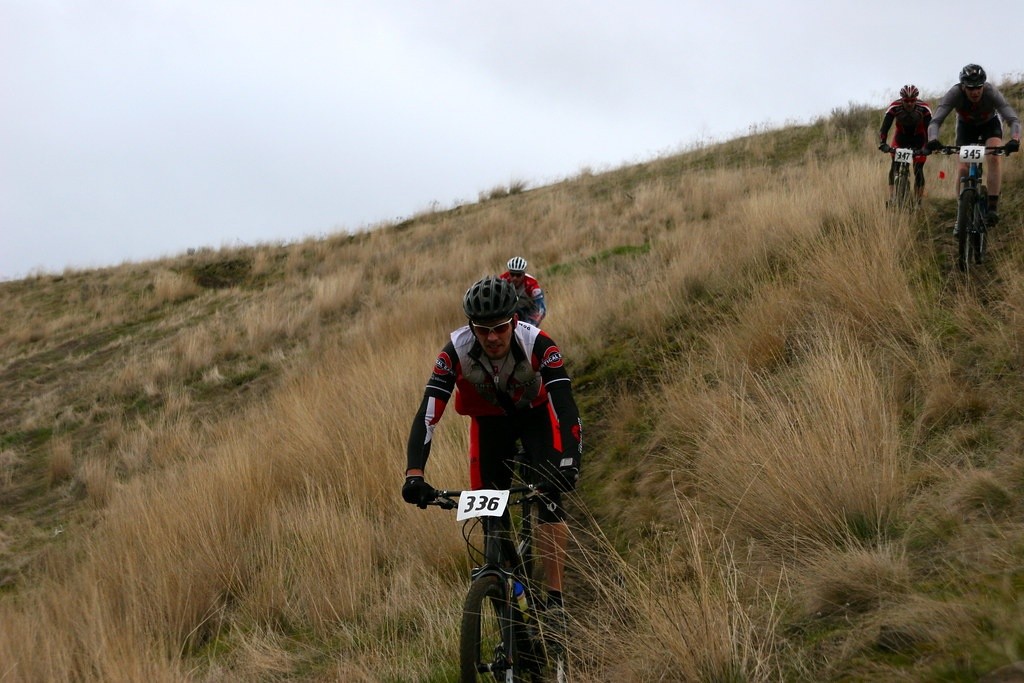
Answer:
[987,209,999,227]
[953,221,960,238]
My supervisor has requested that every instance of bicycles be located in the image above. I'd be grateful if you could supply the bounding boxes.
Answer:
[878,143,932,215]
[932,144,1019,273]
[415,457,584,683]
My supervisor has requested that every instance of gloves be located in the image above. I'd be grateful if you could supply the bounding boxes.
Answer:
[553,467,579,492]
[1004,139,1020,157]
[923,148,932,156]
[926,139,943,154]
[402,475,434,509]
[880,142,890,153]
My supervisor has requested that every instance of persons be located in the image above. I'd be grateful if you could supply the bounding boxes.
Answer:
[879,85,933,211]
[401,276,582,655]
[500,256,546,327]
[928,65,1021,240]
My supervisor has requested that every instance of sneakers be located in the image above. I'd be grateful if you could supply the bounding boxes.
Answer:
[544,602,567,641]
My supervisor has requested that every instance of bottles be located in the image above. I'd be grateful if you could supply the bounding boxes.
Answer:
[512,582,533,637]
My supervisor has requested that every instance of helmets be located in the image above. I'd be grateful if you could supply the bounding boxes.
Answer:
[959,64,986,88]
[900,84,919,98]
[463,273,519,322]
[507,257,527,272]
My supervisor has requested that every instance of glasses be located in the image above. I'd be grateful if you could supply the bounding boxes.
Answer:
[509,271,523,278]
[965,86,983,90]
[904,100,916,103]
[472,318,513,335]
